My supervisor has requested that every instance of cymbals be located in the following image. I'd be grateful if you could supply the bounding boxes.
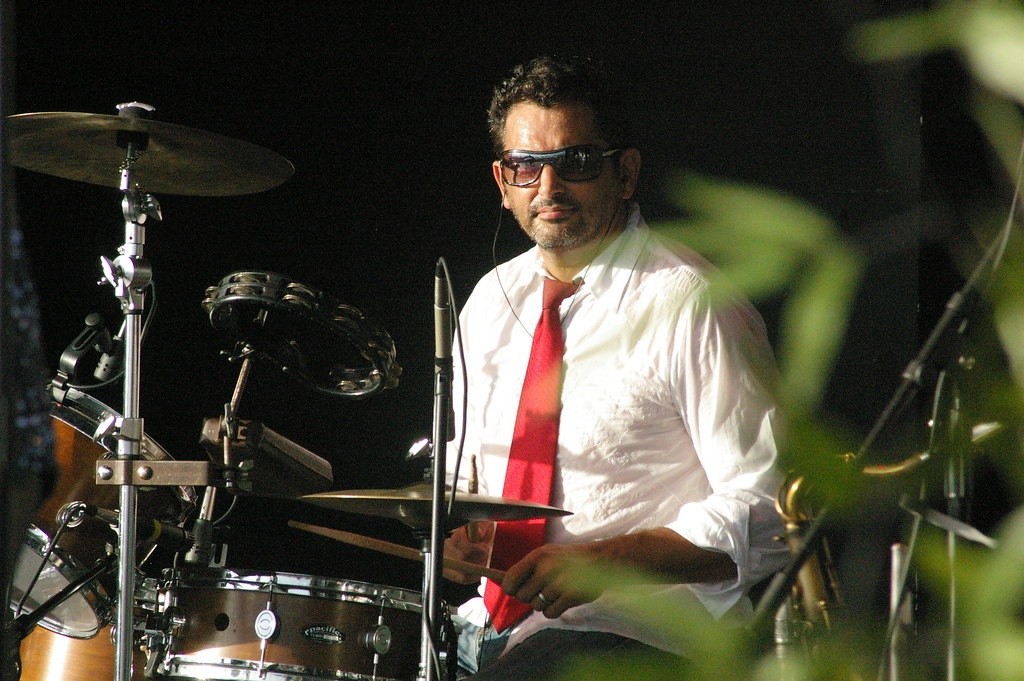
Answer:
[2,101,299,201]
[200,273,404,400]
[292,479,576,524]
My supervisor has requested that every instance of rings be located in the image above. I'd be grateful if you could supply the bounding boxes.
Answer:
[538,592,552,606]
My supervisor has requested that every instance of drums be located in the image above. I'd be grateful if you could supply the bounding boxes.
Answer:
[142,568,465,681]
[1,378,201,641]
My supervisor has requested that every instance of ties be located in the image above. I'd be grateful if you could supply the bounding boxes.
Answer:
[481,281,586,638]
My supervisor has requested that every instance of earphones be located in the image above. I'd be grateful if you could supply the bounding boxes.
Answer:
[621,172,629,185]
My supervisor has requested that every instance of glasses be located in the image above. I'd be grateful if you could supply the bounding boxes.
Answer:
[502,143,623,186]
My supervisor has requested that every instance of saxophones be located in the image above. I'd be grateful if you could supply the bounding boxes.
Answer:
[775,413,1017,680]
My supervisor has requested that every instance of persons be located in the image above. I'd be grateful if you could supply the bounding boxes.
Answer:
[428,56,796,681]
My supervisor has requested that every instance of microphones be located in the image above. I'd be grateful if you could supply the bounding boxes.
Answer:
[185,486,218,567]
[783,475,871,673]
[78,501,196,553]
[431,262,457,445]
